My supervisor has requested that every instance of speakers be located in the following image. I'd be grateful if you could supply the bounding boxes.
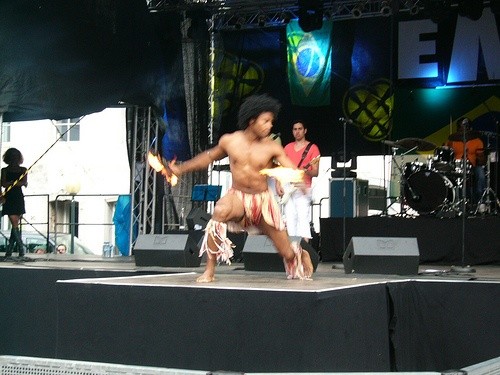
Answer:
[242,235,320,273]
[342,237,420,276]
[187,207,212,230]
[133,233,201,267]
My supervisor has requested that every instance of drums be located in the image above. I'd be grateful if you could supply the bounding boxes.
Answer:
[450,159,472,176]
[398,161,427,184]
[402,169,456,215]
[433,145,456,172]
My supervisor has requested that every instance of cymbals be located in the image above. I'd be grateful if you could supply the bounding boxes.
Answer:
[399,138,436,151]
[448,132,480,143]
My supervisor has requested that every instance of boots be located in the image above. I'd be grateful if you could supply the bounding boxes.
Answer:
[4,228,16,258]
[13,227,24,257]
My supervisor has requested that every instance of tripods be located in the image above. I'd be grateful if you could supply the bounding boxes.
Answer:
[473,136,500,215]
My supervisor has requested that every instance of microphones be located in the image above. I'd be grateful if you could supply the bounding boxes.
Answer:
[338,118,352,124]
[412,196,419,200]
[272,132,281,141]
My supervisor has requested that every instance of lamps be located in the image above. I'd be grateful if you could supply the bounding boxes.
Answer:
[146,0,166,14]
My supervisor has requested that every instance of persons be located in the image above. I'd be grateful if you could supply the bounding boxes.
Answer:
[443,118,488,198]
[56,244,68,254]
[0,148,28,259]
[35,248,46,254]
[172,95,320,281]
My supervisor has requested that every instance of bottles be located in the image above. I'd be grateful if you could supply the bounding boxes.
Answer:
[103,242,111,257]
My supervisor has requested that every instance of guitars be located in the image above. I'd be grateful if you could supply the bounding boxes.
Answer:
[279,154,320,198]
